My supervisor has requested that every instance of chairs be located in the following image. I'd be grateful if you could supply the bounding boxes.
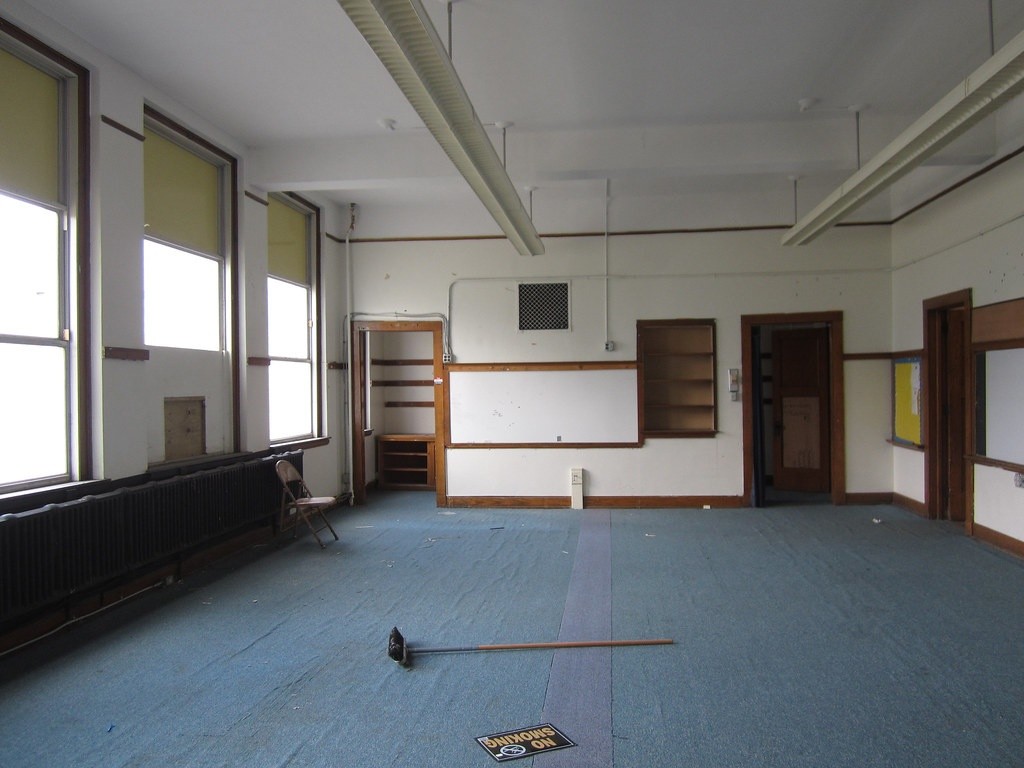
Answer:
[276,459,339,549]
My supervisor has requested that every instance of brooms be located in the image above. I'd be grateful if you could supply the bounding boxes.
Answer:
[385,625,678,667]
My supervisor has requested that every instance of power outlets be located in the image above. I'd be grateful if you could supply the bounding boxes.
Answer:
[728,368,739,392]
[571,468,583,484]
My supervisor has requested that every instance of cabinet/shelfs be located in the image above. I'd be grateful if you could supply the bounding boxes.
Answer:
[375,433,436,491]
